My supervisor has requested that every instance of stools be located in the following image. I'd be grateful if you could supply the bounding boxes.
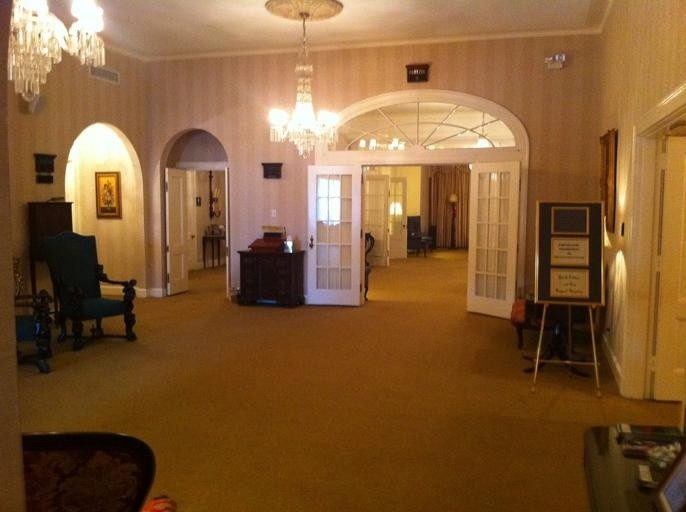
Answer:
[509,295,557,350]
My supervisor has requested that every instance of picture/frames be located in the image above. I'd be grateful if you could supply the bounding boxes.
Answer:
[534,199,610,309]
[94,170,124,220]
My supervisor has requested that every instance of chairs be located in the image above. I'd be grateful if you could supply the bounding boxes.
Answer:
[43,231,137,354]
[22,429,179,511]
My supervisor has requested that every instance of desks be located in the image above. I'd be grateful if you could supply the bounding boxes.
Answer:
[201,234,226,269]
[531,300,610,398]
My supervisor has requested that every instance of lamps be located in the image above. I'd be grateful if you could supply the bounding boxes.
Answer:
[4,0,111,104]
[448,192,459,249]
[263,0,347,163]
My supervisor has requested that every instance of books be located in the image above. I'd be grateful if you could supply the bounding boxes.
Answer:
[616,422,683,489]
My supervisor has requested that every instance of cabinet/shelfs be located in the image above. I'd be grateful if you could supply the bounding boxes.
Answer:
[234,250,308,308]
